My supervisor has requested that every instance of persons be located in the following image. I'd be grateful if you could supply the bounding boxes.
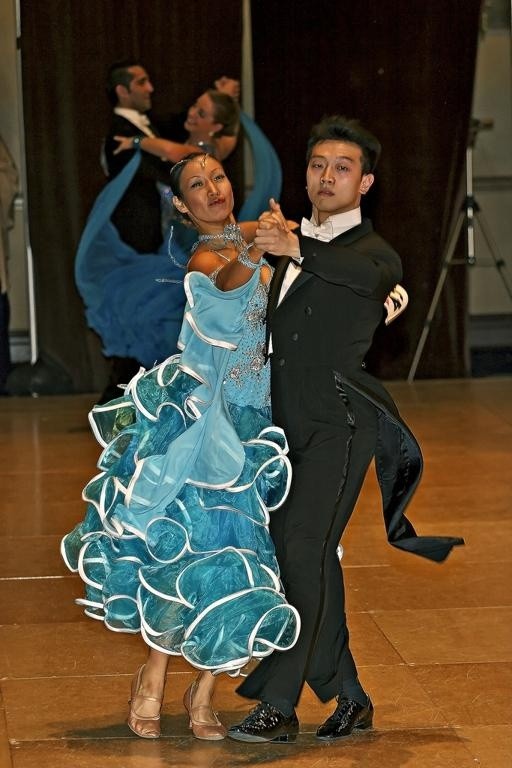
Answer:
[60,148,302,742]
[227,116,466,745]
[92,62,232,406]
[74,78,282,372]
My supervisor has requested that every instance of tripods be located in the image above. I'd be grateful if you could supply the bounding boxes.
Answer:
[406,149,512,384]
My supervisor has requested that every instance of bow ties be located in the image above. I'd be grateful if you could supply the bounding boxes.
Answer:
[300,217,333,241]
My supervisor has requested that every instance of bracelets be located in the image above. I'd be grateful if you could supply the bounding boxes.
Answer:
[238,245,263,269]
[133,134,145,150]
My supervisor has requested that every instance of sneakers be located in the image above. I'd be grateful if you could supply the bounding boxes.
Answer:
[126,664,163,739]
[183,682,227,740]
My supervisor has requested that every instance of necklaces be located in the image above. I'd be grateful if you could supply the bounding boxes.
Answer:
[197,222,243,246]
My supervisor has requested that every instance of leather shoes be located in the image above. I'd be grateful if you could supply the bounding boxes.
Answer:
[316,692,374,740]
[228,704,298,743]
